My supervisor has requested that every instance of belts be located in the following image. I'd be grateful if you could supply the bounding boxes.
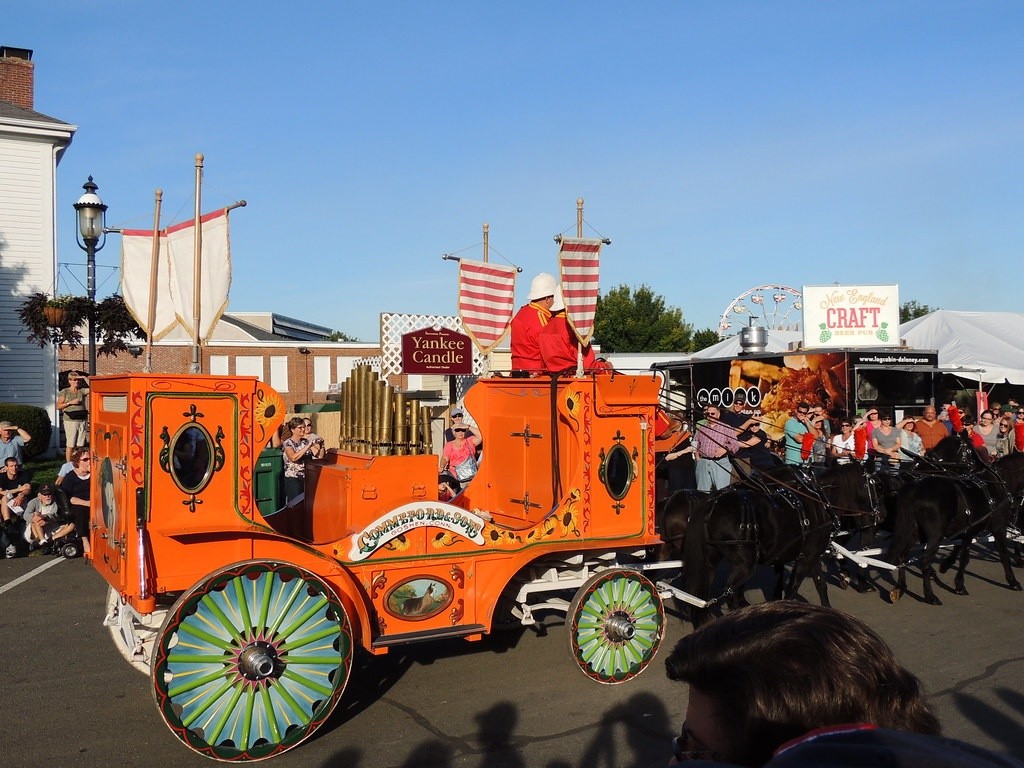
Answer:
[701,452,727,461]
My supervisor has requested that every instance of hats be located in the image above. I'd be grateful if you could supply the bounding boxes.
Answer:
[451,408,463,416]
[36,483,51,495]
[733,394,746,404]
[1000,404,1018,414]
[438,469,459,489]
[527,273,565,312]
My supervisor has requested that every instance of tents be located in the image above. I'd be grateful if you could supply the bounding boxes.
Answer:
[681,310,1024,396]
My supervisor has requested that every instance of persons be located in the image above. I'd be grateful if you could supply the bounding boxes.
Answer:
[176,431,208,489]
[538,284,683,440]
[271,417,326,522]
[510,276,558,378]
[0,371,91,558]
[665,598,944,768]
[655,402,1024,493]
[439,408,482,501]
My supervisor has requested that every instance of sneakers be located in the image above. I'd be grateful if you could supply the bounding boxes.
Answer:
[660,420,682,439]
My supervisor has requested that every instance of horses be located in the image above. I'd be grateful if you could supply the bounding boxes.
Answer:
[658,427,1024,632]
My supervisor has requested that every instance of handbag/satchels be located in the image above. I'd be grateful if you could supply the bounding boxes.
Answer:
[455,453,478,483]
[64,409,89,419]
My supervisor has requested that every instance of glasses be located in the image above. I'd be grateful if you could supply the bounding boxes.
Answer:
[840,413,890,427]
[749,423,760,427]
[959,411,965,415]
[982,417,992,421]
[80,458,90,462]
[452,414,462,418]
[454,428,467,433]
[297,424,312,429]
[1018,411,1024,415]
[703,408,717,416]
[999,422,1008,429]
[993,408,1000,411]
[735,402,744,406]
[672,737,729,762]
[798,410,807,415]
[962,423,972,426]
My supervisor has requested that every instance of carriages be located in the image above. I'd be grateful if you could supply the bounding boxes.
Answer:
[102,318,1024,763]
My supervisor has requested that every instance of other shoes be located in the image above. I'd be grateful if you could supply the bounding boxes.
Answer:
[5,522,53,545]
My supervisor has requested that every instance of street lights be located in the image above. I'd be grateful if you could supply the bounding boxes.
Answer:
[67,176,109,379]
